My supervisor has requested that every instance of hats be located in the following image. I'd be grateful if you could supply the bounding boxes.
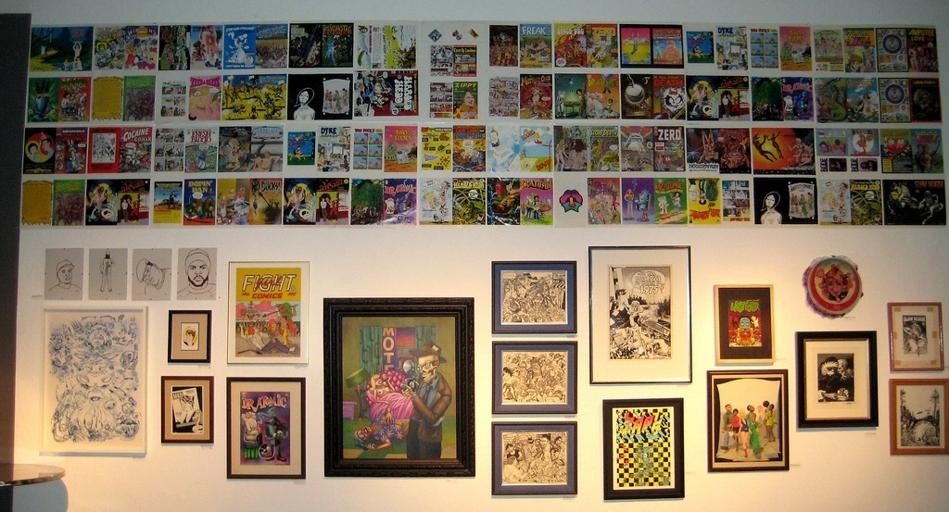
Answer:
[408,342,442,358]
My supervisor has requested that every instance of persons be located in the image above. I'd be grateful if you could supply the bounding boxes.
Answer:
[177,250,216,300]
[135,257,171,299]
[183,329,196,348]
[720,402,777,458]
[46,260,82,300]
[817,264,853,302]
[99,251,115,293]
[174,395,196,424]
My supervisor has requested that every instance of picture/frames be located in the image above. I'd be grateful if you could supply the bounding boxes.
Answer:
[886,302,944,372]
[490,422,578,495]
[227,260,308,365]
[323,297,476,478]
[804,256,864,317]
[714,284,777,366]
[491,260,577,335]
[168,309,211,362]
[160,375,214,443]
[492,340,578,414]
[707,370,789,472]
[888,379,947,454]
[602,398,684,500]
[42,307,145,458]
[226,376,306,479]
[588,246,692,384]
[796,330,879,429]
